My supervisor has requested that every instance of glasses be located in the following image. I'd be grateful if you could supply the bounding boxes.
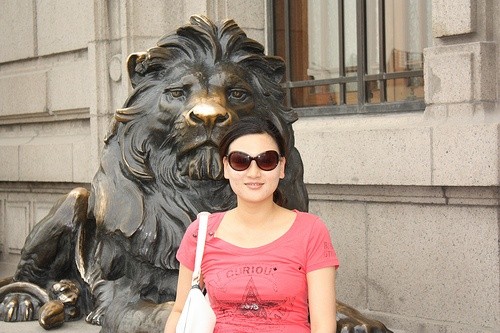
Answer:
[224,150,281,171]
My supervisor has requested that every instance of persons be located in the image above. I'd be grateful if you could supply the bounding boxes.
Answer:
[163,119,340,333]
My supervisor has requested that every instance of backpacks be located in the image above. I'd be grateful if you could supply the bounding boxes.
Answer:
[175,211,217,333]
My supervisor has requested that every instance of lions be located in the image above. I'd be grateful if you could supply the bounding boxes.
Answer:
[0,15,392,333]
